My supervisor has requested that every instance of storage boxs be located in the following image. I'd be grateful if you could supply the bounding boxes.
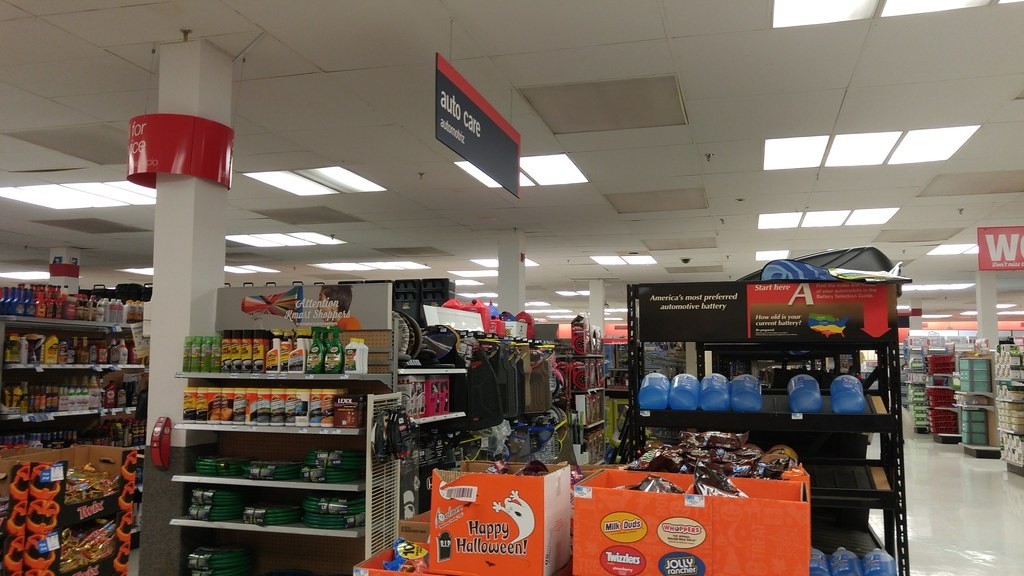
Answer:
[923,356,991,445]
[343,277,455,325]
[428,459,570,576]
[502,321,527,338]
[572,470,812,576]
[352,542,570,576]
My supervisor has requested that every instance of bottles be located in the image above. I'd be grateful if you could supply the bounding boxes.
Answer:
[0,282,147,449]
[181,325,368,428]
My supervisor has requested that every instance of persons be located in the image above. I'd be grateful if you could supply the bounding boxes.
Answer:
[317,285,362,330]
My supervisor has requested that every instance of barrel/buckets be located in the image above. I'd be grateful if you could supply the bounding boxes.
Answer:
[700,373,731,412]
[730,374,764,412]
[788,375,822,412]
[637,372,670,409]
[863,547,897,576]
[669,374,700,410]
[830,375,865,414]
[809,546,831,576]
[829,547,863,576]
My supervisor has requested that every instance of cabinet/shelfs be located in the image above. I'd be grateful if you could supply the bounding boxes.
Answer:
[2,319,1024,576]
